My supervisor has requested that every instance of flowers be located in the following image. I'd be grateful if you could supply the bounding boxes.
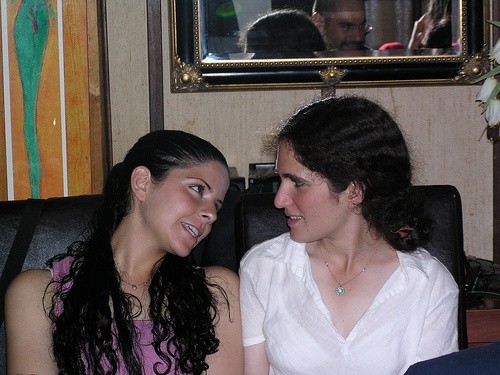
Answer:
[469,20,500,128]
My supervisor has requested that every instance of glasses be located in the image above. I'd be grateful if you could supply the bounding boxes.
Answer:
[341,22,373,33]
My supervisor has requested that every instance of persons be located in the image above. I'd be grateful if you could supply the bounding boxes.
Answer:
[311,1,373,53]
[238,96,460,375]
[245,11,326,59]
[407,1,454,48]
[7,130,243,375]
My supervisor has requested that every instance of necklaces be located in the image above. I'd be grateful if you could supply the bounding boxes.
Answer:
[316,240,383,296]
[121,279,148,290]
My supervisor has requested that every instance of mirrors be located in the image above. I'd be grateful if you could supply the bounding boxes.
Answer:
[166,0,487,94]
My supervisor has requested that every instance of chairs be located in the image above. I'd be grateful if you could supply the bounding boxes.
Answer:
[1,178,465,375]
